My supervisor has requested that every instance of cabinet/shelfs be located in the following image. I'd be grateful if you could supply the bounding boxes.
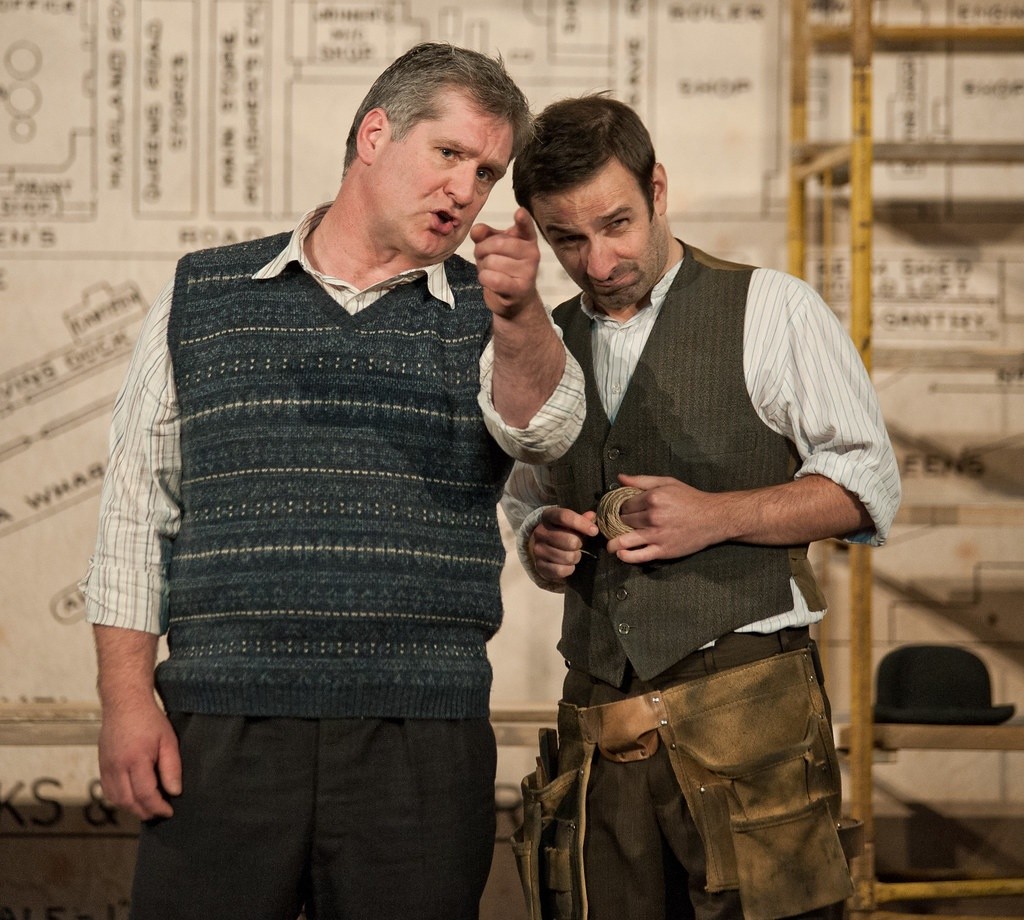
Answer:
[786,0,1024,920]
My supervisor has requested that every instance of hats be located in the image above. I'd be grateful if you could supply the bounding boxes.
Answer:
[875,643,1015,725]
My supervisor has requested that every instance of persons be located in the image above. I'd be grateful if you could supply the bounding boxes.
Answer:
[501,95,903,920]
[79,40,588,919]
